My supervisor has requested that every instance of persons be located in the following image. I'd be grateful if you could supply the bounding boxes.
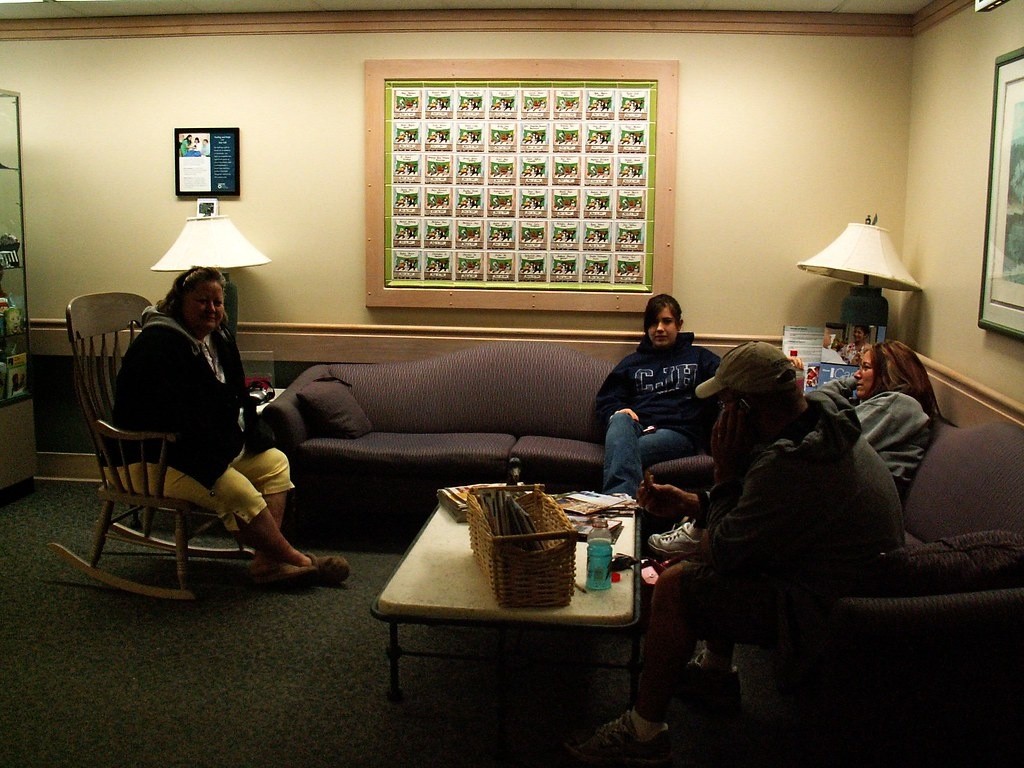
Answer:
[111,268,351,582]
[595,294,721,499]
[647,340,936,556]
[823,325,872,367]
[562,341,906,766]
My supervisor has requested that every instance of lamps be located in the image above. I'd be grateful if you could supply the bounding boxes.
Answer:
[149,215,272,342]
[796,222,922,326]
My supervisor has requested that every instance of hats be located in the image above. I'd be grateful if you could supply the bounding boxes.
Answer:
[695,341,798,399]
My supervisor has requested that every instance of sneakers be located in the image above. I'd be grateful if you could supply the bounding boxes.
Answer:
[561,710,673,764]
[647,520,702,556]
[680,651,741,708]
[247,552,350,584]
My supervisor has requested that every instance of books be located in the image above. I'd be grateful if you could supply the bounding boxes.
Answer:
[437,483,507,523]
[555,491,636,518]
[6,353,27,399]
[480,489,545,550]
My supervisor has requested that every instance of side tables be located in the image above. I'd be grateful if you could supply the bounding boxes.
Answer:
[239,388,285,441]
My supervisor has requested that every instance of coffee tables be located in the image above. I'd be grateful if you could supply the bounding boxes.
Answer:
[370,492,643,758]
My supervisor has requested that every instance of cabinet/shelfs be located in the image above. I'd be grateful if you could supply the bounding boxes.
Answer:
[0,90,38,506]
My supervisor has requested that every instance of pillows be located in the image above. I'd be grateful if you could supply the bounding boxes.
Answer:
[296,377,372,439]
[869,530,1023,596]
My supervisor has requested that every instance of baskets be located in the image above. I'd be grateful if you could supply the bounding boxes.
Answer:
[469,483,578,609]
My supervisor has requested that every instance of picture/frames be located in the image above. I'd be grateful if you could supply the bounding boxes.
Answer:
[978,45,1024,342]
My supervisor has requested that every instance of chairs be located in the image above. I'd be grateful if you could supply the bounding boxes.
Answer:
[46,291,255,600]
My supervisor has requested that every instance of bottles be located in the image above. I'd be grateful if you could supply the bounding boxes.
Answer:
[585,519,612,590]
[789,350,805,395]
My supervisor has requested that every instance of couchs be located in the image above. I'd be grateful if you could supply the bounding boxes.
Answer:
[259,343,1024,768]
